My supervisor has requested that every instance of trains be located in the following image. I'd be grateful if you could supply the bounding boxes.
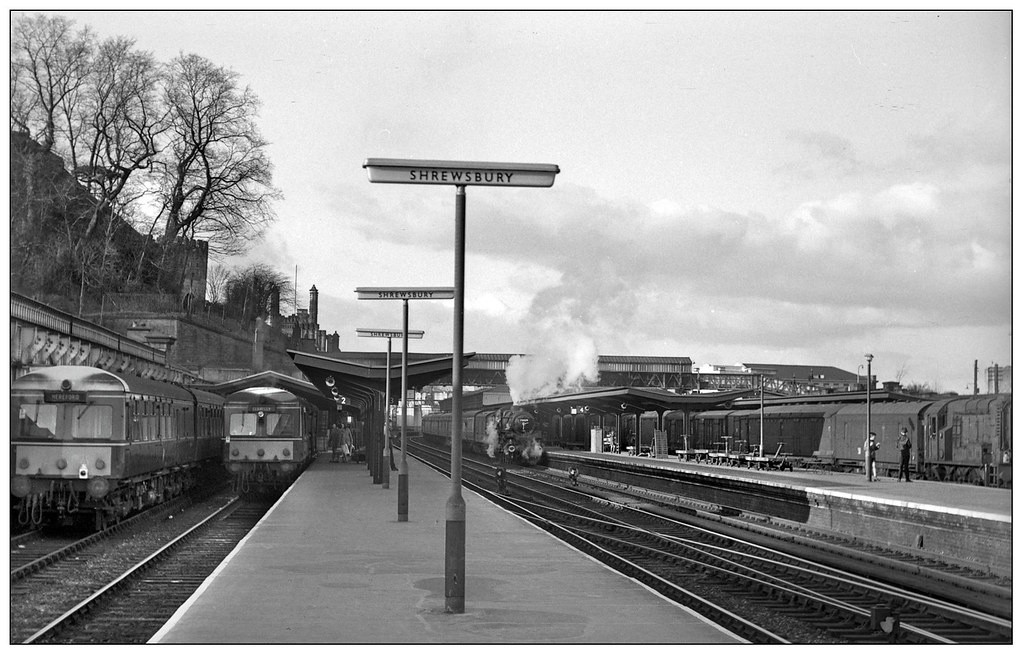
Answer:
[10,364,225,538]
[543,392,1012,490]
[221,386,319,497]
[420,405,524,458]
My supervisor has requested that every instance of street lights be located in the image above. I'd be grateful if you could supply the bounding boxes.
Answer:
[864,353,874,481]
[856,364,864,383]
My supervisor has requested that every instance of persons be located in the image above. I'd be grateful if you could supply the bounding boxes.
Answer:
[327,424,354,461]
[896,427,912,483]
[865,432,880,482]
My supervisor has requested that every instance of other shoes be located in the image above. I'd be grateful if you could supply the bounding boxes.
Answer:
[906,480,913,482]
[874,478,880,481]
[897,479,901,482]
